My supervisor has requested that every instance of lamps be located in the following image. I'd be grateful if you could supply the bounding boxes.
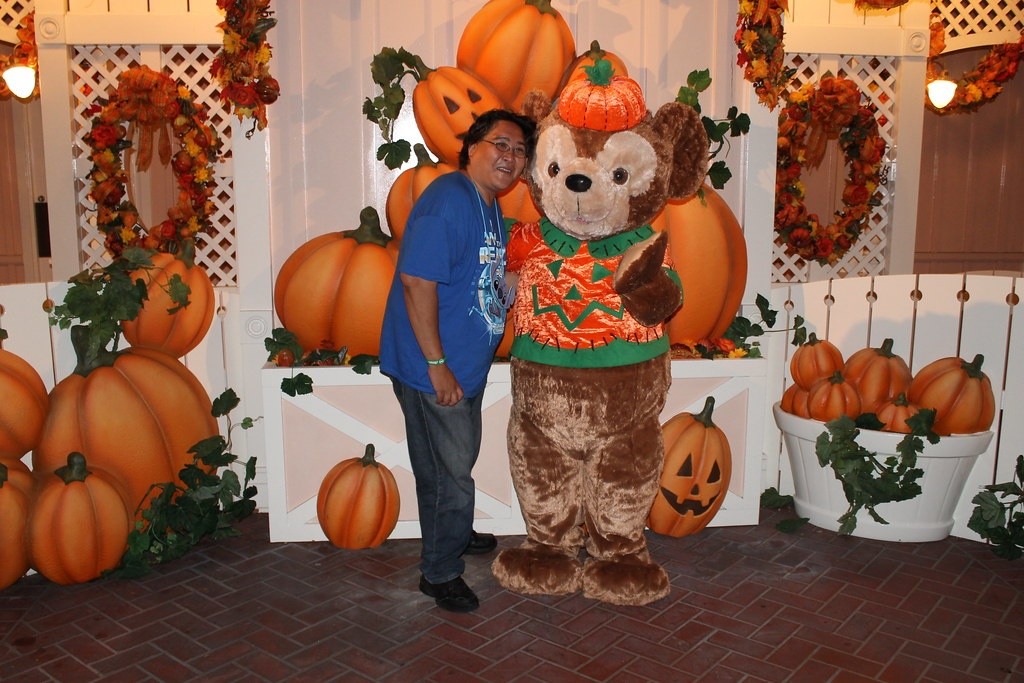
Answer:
[3,41,37,99]
[927,60,957,108]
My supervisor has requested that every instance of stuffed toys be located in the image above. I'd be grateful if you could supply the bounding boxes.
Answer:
[490,61,706,606]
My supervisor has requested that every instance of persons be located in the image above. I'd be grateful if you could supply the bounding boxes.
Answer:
[379,110,536,613]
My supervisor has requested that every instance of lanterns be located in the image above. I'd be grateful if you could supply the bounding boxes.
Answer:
[649,397,732,536]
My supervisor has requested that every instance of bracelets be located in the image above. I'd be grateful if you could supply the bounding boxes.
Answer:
[427,358,445,365]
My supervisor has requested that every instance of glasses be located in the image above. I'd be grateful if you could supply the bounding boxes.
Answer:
[482,140,528,159]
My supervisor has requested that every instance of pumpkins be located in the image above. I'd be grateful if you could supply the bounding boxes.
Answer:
[1,237,222,587]
[779,332,996,437]
[273,0,750,350]
[316,444,401,549]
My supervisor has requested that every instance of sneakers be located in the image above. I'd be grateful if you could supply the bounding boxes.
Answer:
[419,574,480,613]
[464,529,498,555]
